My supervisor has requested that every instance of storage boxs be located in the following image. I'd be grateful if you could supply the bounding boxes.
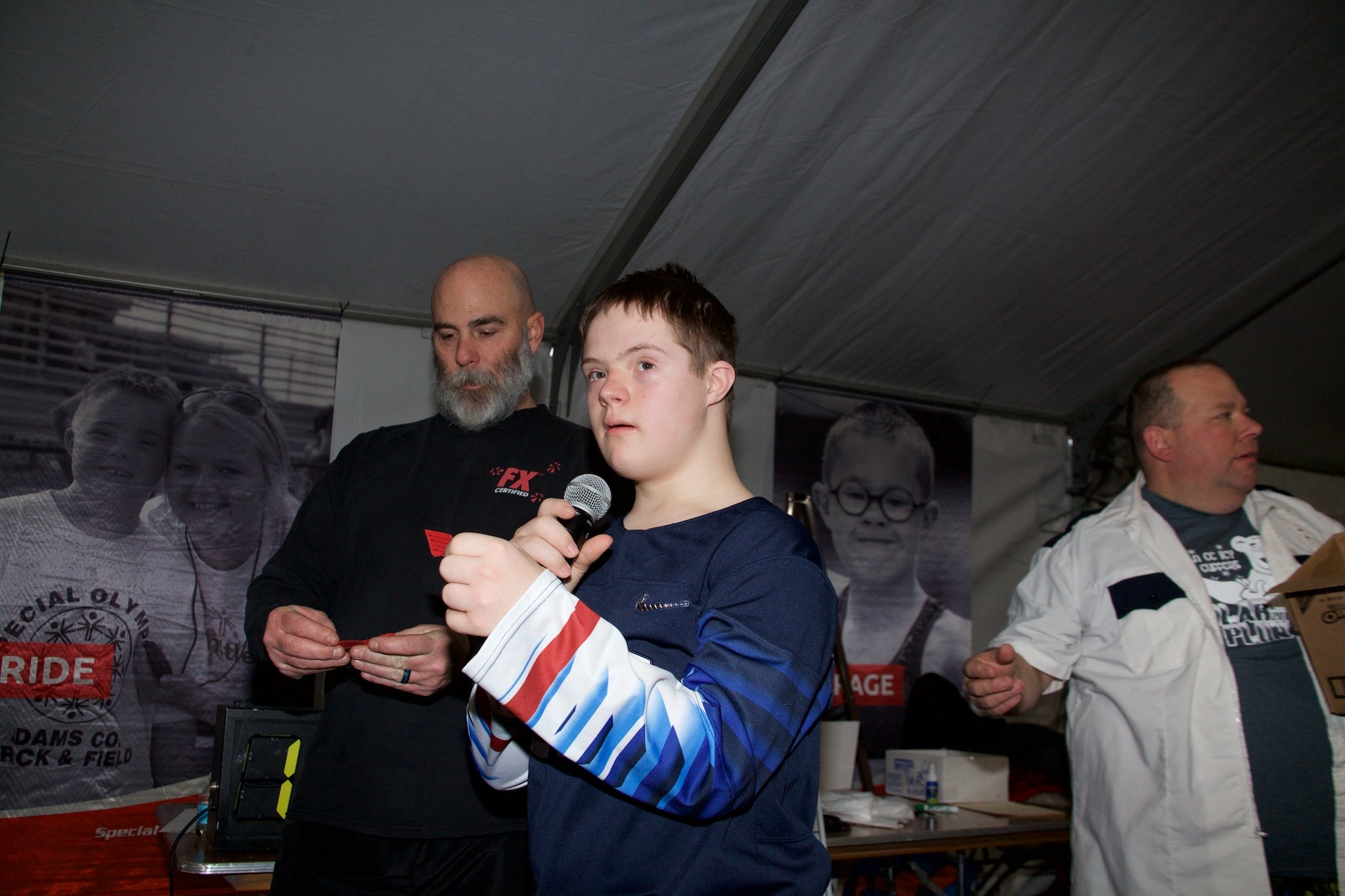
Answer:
[1264,530,1345,714]
[885,747,1009,806]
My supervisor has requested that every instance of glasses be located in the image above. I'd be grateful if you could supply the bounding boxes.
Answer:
[176,389,269,420]
[831,479,925,523]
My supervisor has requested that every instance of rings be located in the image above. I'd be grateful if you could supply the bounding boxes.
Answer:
[400,670,411,685]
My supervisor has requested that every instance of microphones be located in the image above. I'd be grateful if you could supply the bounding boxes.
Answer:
[554,473,612,584]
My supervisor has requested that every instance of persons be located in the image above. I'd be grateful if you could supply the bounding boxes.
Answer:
[243,254,634,896]
[961,359,1345,896]
[809,410,974,759]
[439,261,838,896]
[139,382,305,772]
[0,363,210,808]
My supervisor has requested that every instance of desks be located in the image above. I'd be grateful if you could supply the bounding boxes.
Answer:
[822,784,1071,896]
[157,802,277,875]
